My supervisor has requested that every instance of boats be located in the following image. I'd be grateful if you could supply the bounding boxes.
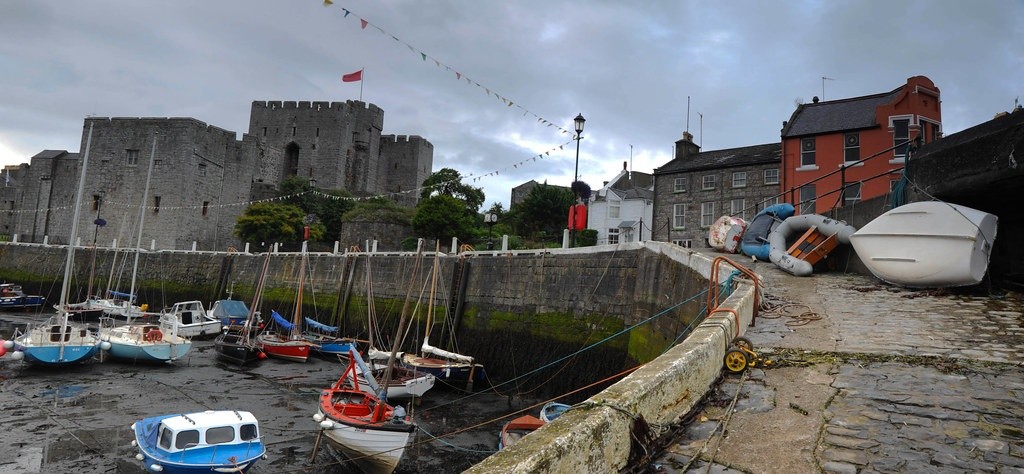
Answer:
[158,300,264,338]
[0,283,45,308]
[849,201,999,287]
[742,203,795,262]
[708,215,747,254]
[318,349,419,474]
[499,414,547,451]
[769,213,857,277]
[133,410,266,474]
[540,401,572,423]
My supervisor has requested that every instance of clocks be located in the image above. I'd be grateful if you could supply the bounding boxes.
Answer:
[484,213,491,222]
[492,214,497,222]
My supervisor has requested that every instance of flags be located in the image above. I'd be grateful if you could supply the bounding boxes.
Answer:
[342,70,361,82]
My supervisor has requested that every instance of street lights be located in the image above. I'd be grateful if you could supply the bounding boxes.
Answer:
[571,112,586,247]
[94,189,105,245]
[483,208,498,250]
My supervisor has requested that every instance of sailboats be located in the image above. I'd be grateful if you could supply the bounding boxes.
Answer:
[256,240,320,363]
[271,248,359,355]
[14,121,192,364]
[336,261,435,399]
[214,244,273,365]
[402,238,484,383]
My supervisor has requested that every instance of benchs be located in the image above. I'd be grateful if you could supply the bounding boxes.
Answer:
[334,403,374,416]
